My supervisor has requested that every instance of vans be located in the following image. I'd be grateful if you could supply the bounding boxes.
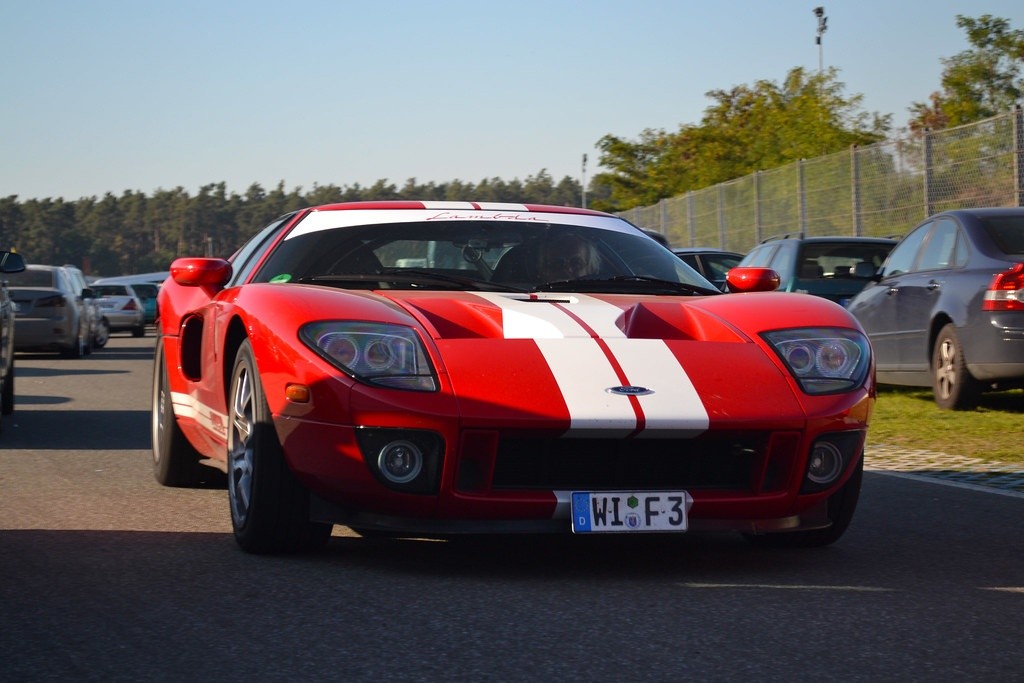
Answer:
[92,270,171,325]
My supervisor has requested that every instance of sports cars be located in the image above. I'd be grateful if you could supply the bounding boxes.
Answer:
[152,201,874,551]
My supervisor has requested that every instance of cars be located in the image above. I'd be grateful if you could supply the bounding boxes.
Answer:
[847,208,1024,410]
[0,250,26,412]
[671,247,746,294]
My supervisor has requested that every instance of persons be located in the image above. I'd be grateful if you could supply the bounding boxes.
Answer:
[534,232,600,286]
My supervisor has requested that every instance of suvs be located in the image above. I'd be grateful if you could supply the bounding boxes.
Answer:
[1,263,110,358]
[89,285,146,338]
[724,233,903,317]
